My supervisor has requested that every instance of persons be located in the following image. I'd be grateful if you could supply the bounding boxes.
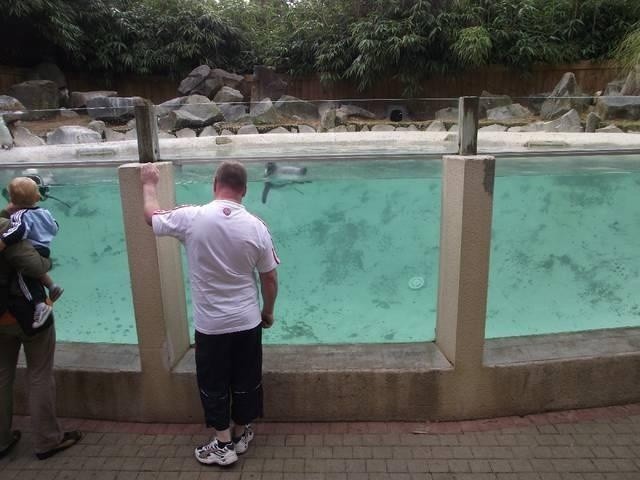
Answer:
[0,176,59,329]
[0,203,85,460]
[139,161,282,467]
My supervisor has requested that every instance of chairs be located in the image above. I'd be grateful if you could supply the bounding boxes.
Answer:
[49,285,64,302]
[231,422,254,454]
[32,304,53,328]
[194,437,239,466]
[36,429,82,460]
[0,430,22,454]
